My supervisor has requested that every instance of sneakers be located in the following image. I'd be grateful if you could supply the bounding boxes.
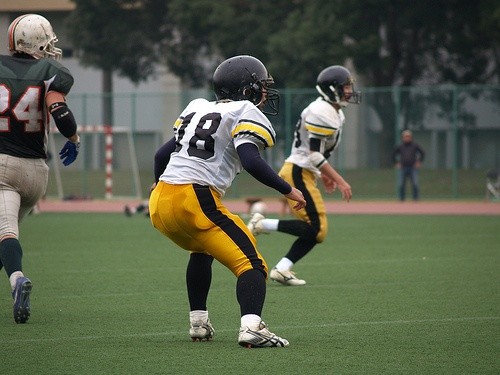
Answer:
[238,320,289,349]
[246,213,264,236]
[11,277,33,324]
[269,266,306,285]
[189,318,215,342]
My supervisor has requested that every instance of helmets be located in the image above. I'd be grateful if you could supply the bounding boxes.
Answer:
[316,65,352,101]
[8,14,54,60]
[212,55,268,105]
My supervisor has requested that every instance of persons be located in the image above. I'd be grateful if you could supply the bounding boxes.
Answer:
[392,129,425,201]
[0,12,82,326]
[247,65,354,286]
[149,54,306,346]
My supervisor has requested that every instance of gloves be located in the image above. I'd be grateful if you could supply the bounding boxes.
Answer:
[59,136,81,166]
